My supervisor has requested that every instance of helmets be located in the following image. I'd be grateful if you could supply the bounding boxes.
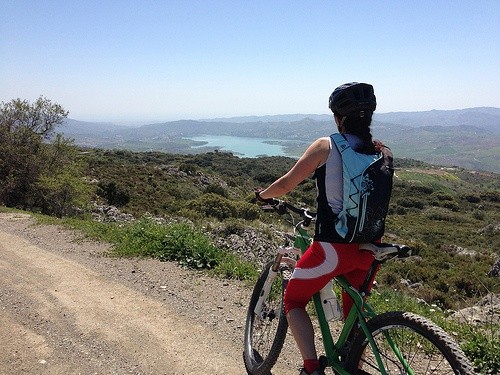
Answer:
[328,81,378,115]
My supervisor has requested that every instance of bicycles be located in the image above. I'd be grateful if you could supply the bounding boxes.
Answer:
[244,192,473,375]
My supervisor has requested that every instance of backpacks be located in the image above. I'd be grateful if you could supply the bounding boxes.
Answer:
[328,132,395,245]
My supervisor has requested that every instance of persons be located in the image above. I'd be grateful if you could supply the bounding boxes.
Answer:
[255,81,393,375]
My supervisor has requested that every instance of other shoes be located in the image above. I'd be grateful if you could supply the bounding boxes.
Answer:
[298,368,326,375]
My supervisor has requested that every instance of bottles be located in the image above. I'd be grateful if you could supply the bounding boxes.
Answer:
[319,279,342,321]
